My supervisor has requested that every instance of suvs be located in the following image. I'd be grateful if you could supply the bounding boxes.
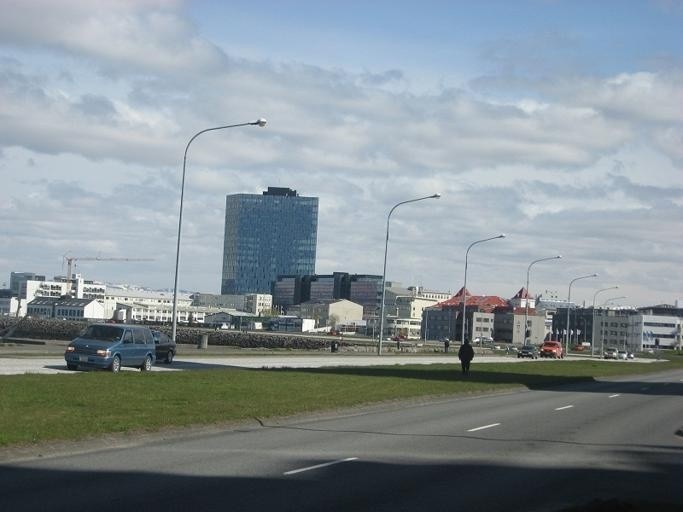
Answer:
[540,341,564,359]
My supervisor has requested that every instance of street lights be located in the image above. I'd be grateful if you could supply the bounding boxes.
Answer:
[564,273,597,354]
[170,118,266,343]
[591,285,619,357]
[600,296,629,359]
[377,192,441,356]
[522,254,561,346]
[460,233,506,345]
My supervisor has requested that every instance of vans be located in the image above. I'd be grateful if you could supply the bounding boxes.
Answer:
[65,322,155,373]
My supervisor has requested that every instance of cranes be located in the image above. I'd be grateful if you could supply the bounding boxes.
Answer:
[61,252,154,299]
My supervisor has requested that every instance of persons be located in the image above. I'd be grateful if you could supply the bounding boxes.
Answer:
[458,338,475,373]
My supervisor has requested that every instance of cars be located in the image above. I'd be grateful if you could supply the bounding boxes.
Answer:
[575,342,633,360]
[517,345,538,359]
[151,330,175,366]
[437,336,493,344]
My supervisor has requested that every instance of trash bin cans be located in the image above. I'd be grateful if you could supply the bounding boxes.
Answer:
[331,341,339,352]
[197,334,208,349]
